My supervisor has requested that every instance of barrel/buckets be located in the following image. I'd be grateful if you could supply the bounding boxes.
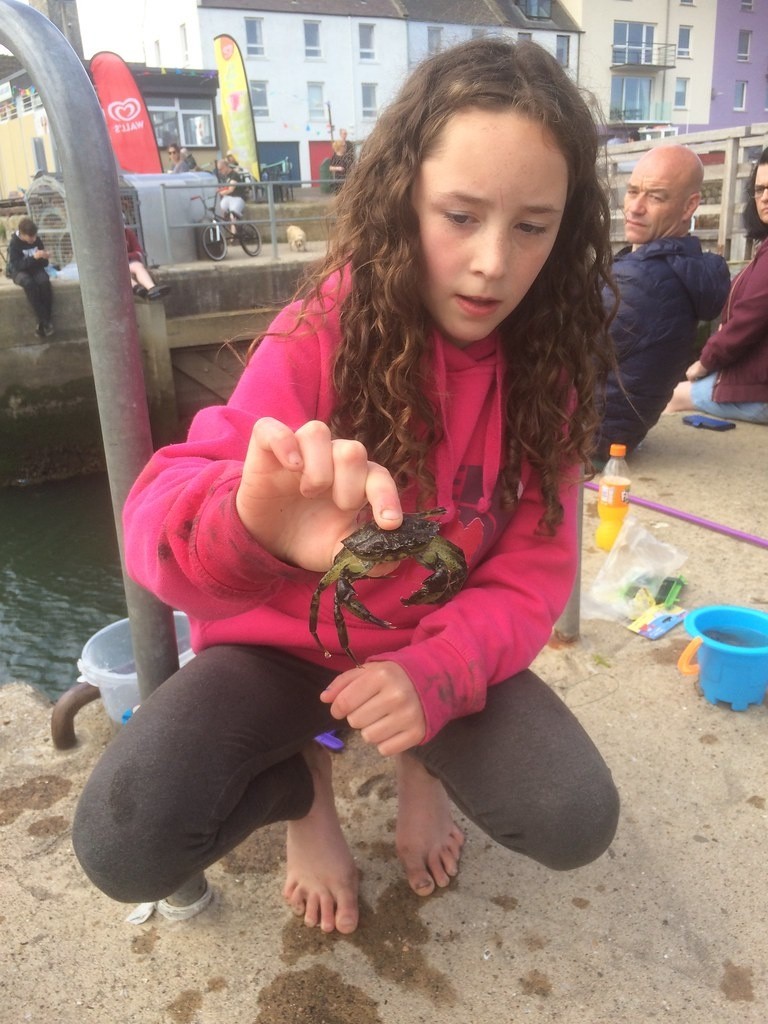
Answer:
[675,604,768,712]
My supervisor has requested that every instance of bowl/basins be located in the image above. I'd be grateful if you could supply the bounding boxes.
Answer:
[82,610,198,720]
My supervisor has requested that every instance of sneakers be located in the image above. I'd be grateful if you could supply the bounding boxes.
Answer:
[35,324,43,338]
[147,285,170,300]
[133,283,146,299]
[43,323,53,336]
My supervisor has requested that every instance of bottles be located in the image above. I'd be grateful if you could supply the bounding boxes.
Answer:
[595,443,631,552]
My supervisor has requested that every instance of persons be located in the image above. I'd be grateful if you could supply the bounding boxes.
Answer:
[70,38,636,938]
[117,210,172,300]
[329,139,352,193]
[338,129,353,153]
[179,147,197,170]
[166,144,188,173]
[214,159,248,243]
[4,219,57,338]
[685,147,767,427]
[226,155,244,173]
[581,140,733,476]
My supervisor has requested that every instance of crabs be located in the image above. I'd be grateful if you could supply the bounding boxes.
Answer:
[309,507,468,669]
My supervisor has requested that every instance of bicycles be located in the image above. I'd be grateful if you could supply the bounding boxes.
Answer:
[191,186,262,262]
